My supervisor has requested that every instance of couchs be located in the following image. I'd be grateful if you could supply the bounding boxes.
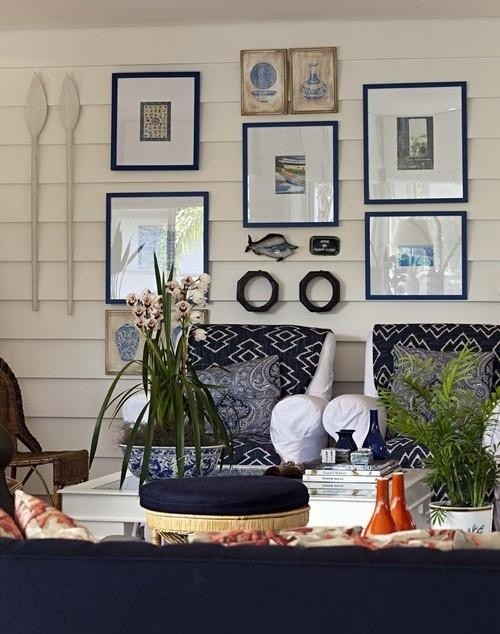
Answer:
[0,541,500,634]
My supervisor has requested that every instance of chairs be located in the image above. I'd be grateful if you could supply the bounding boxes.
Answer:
[323,324,500,470]
[121,323,335,469]
[0,357,88,512]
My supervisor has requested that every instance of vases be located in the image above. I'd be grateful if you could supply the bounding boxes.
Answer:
[390,470,416,532]
[118,440,223,482]
[362,477,398,539]
[125,274,212,345]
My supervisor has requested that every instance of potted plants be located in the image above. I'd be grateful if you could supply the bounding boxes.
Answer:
[376,341,494,533]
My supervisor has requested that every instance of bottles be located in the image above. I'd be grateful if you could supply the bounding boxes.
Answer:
[300,64,328,102]
[362,409,387,461]
[364,473,416,537]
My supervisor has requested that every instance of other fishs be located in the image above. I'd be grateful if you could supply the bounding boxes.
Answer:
[245,234,298,262]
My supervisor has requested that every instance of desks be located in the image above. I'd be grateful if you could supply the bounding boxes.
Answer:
[56,468,432,540]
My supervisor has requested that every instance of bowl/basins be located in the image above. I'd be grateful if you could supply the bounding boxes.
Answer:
[250,91,278,102]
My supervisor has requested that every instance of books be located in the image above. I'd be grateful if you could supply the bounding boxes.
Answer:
[302,459,403,501]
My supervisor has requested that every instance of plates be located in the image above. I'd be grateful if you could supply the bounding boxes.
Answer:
[251,63,276,89]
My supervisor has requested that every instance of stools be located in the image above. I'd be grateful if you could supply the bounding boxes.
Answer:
[140,475,310,546]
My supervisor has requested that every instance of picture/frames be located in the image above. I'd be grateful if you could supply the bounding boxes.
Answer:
[104,309,208,376]
[240,49,287,116]
[362,81,468,204]
[365,211,467,300]
[288,47,338,114]
[106,191,209,304]
[242,120,339,228]
[111,71,200,171]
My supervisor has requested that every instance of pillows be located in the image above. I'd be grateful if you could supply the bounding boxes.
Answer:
[392,343,494,423]
[13,487,98,544]
[186,354,280,439]
